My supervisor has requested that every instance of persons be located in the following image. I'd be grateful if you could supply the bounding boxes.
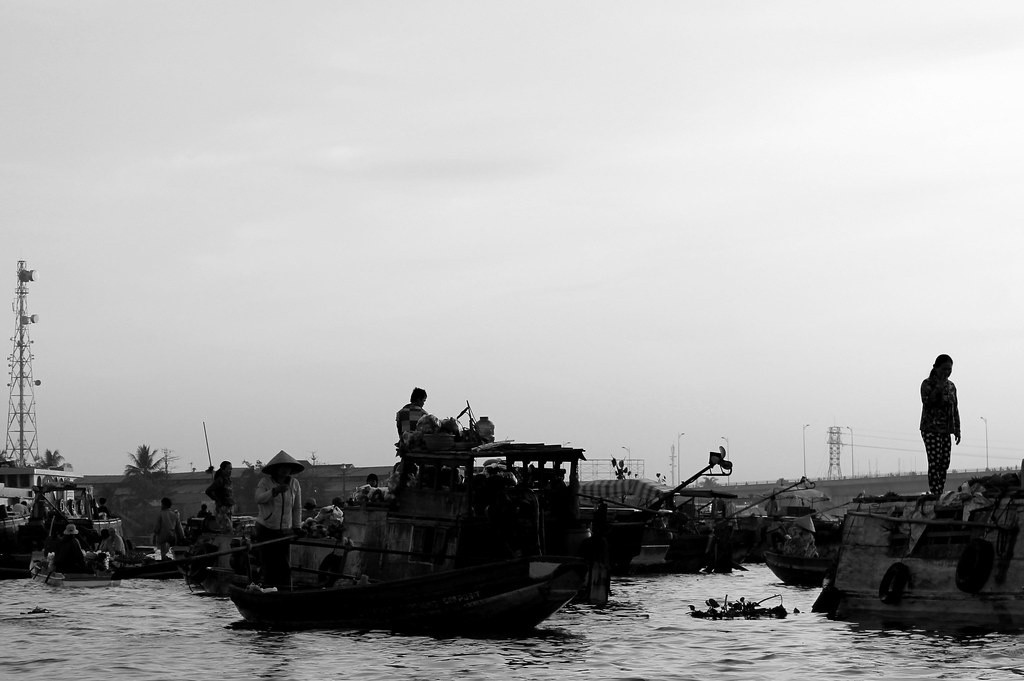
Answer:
[98,498,118,518]
[13,501,29,515]
[919,354,961,495]
[396,388,428,438]
[42,524,126,573]
[153,497,186,560]
[255,450,307,579]
[333,497,342,507]
[205,461,235,534]
[366,474,378,488]
[197,504,211,518]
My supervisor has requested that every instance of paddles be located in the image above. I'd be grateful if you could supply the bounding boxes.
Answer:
[148,534,300,566]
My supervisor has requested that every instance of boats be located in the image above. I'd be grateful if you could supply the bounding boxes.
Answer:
[227,401,588,639]
[0,465,263,584]
[582,446,1024,635]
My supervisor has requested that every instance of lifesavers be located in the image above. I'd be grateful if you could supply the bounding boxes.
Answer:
[879,561,909,604]
[955,536,995,595]
[318,553,340,588]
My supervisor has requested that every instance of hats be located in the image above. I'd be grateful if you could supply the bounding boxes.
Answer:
[63,524,79,535]
[306,498,316,505]
[262,450,306,475]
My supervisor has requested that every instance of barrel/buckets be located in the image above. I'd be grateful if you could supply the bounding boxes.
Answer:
[567,527,592,557]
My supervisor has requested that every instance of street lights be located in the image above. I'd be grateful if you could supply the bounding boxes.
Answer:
[980,416,990,467]
[622,446,631,473]
[803,424,810,478]
[677,432,686,485]
[846,425,854,479]
[721,436,730,487]
